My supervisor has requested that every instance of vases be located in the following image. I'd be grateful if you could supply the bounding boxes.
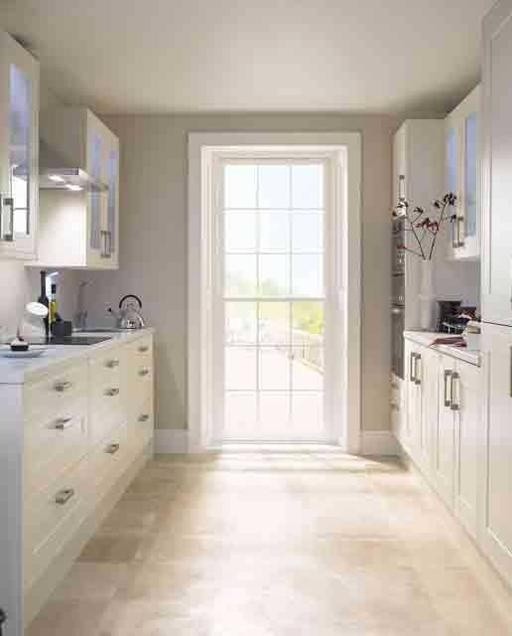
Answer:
[421,259,435,330]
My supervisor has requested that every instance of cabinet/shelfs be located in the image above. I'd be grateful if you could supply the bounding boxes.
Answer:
[482,1,512,330]
[402,331,482,540]
[393,119,446,453]
[24,106,121,272]
[0,326,158,636]
[446,82,481,263]
[0,30,41,260]
[481,322,511,596]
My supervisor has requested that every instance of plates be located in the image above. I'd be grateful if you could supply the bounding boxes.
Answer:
[1,349,45,358]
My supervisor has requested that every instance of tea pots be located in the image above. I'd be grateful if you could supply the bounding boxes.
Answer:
[106,294,146,329]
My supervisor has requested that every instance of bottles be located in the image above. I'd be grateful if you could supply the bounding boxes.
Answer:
[50,285,57,322]
[38,270,49,338]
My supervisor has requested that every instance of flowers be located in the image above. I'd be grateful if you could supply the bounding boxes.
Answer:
[390,193,456,259]
